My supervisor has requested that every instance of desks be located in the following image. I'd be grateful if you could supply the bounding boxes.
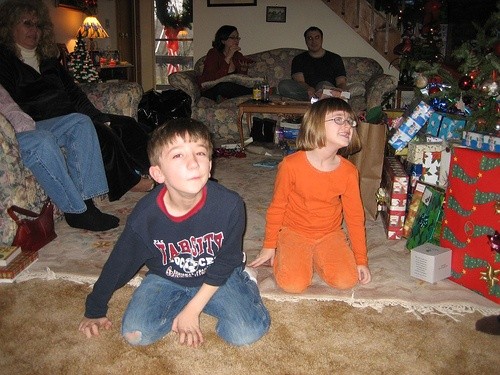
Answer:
[96,63,135,81]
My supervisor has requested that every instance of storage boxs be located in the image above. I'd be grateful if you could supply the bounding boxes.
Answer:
[377,100,500,304]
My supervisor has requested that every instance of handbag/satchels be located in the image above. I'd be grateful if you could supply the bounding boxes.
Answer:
[7,197,62,249]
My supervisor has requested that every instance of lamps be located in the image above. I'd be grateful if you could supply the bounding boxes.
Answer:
[75,16,109,50]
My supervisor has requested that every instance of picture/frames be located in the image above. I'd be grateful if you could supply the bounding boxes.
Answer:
[207,0,257,7]
[265,6,287,23]
[55,0,93,16]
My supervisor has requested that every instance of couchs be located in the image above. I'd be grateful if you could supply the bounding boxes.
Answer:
[168,47,398,142]
[0,79,144,246]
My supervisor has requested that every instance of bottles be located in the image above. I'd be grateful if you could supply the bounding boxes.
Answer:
[252,79,270,105]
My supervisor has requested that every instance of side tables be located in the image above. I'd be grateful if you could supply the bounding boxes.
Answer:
[396,80,415,110]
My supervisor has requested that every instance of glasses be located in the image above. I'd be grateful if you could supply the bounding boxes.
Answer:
[306,35,321,41]
[325,117,359,128]
[20,18,44,30]
[227,36,243,41]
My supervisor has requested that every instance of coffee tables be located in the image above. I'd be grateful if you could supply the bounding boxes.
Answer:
[236,98,312,153]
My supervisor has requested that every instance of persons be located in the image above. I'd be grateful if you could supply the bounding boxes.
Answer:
[78,116,272,345]
[200,26,253,103]
[246,97,372,293]
[278,26,346,102]
[0,1,120,230]
